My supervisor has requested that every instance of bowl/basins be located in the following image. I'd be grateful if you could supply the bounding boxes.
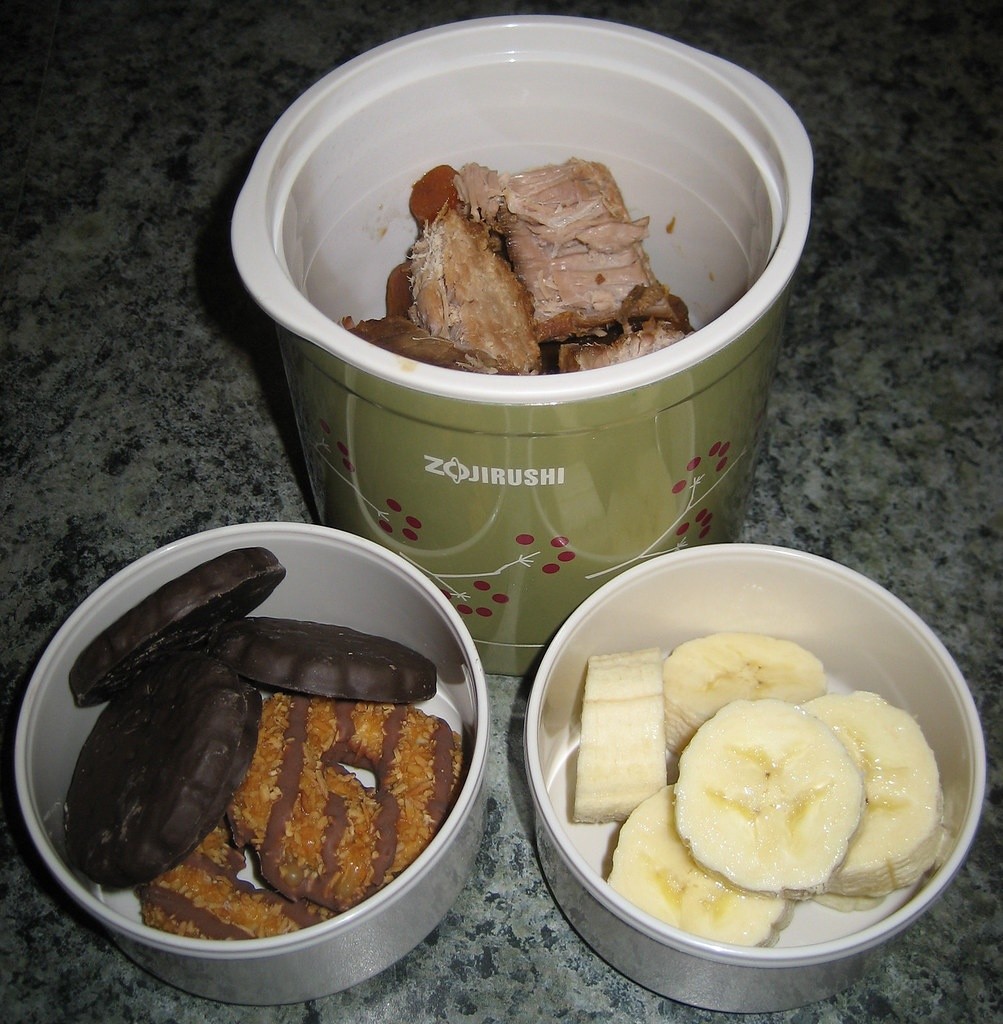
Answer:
[15,523,490,1005]
[520,544,987,1014]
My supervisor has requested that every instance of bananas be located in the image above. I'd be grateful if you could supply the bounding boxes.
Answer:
[574,632,947,949]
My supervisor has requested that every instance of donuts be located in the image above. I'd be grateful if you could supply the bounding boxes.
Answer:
[62,546,465,941]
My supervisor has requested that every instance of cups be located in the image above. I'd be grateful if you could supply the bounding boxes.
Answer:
[229,14,813,677]
